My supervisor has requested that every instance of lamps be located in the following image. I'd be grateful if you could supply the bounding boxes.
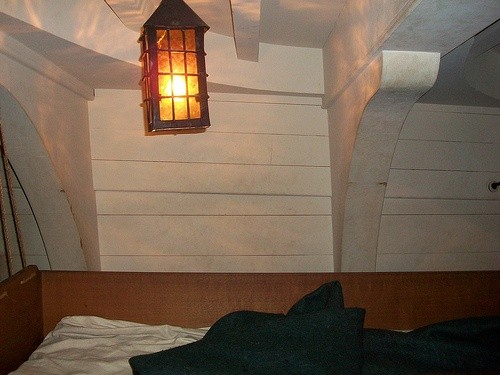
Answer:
[137,0,212,137]
